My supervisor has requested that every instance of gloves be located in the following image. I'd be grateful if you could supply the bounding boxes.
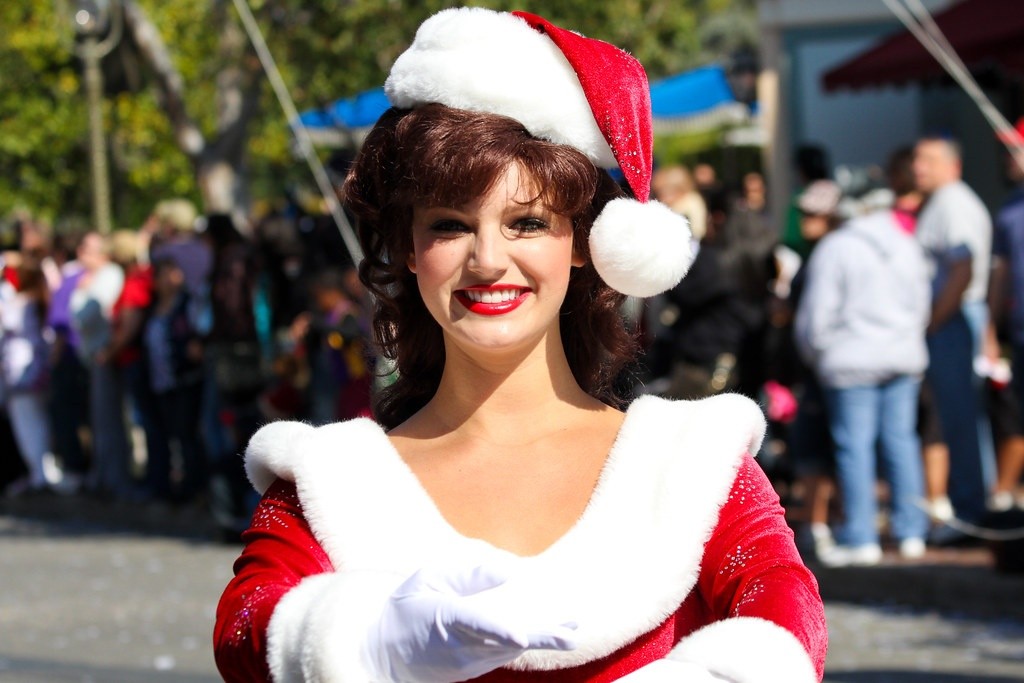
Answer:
[375,562,577,683]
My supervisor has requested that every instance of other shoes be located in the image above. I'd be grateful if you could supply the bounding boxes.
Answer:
[823,542,881,568]
[899,538,925,559]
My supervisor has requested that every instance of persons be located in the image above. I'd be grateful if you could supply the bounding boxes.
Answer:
[598,119,1024,567]
[212,7,830,683]
[0,156,403,525]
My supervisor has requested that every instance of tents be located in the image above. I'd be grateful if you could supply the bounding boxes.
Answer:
[288,61,746,152]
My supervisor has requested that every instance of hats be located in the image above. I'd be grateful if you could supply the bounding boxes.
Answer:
[794,180,840,216]
[383,5,694,297]
